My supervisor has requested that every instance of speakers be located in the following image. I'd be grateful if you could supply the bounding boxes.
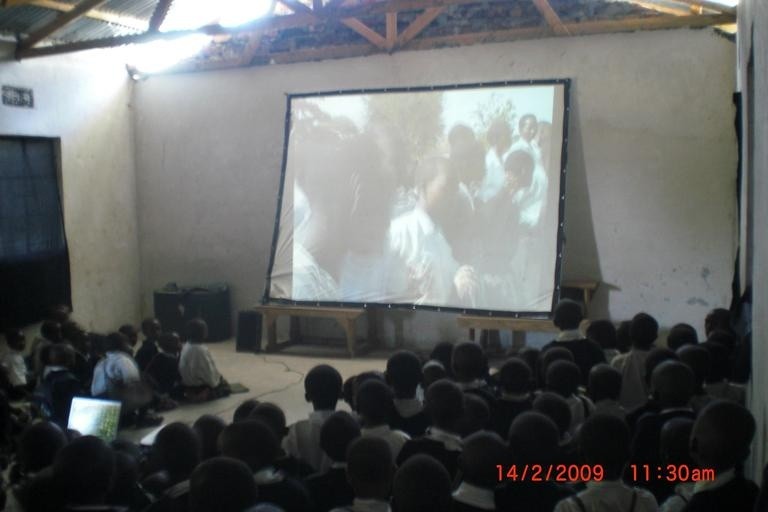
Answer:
[235,311,263,353]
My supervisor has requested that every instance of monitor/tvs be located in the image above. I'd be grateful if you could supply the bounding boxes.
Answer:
[66,396,123,447]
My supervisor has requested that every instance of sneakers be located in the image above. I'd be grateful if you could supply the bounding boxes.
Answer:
[136,412,164,429]
[156,397,178,410]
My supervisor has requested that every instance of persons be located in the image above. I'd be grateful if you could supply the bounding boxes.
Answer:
[1,298,764,511]
[291,102,552,311]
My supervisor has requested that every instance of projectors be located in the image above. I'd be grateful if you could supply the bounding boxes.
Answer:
[140,424,167,446]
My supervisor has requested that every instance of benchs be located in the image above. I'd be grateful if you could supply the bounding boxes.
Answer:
[457,281,597,361]
[253,301,372,358]
